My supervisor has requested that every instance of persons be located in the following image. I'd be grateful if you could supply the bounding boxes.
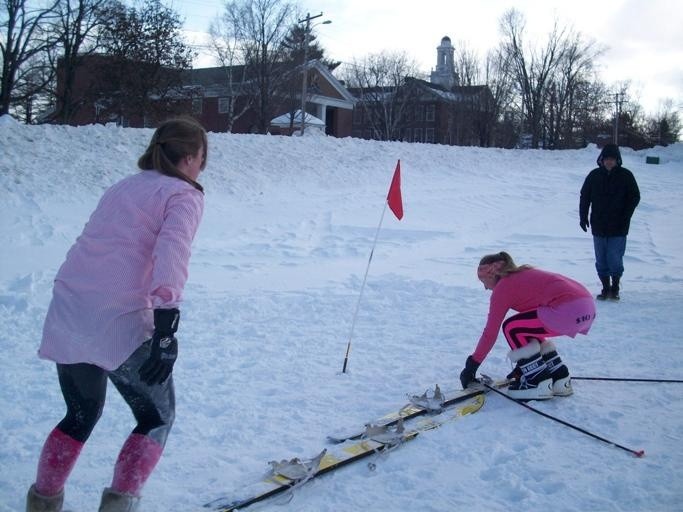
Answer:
[576,143,642,302]
[456,251,598,403]
[24,113,209,511]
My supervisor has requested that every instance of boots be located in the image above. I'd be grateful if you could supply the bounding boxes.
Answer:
[597,274,622,301]
[99,486,142,512]
[24,482,67,511]
[506,338,573,401]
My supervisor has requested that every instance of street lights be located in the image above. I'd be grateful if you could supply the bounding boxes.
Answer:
[300,19,332,136]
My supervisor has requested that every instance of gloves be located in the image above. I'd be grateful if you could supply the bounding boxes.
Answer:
[459,355,481,390]
[580,219,590,232]
[137,305,183,386]
